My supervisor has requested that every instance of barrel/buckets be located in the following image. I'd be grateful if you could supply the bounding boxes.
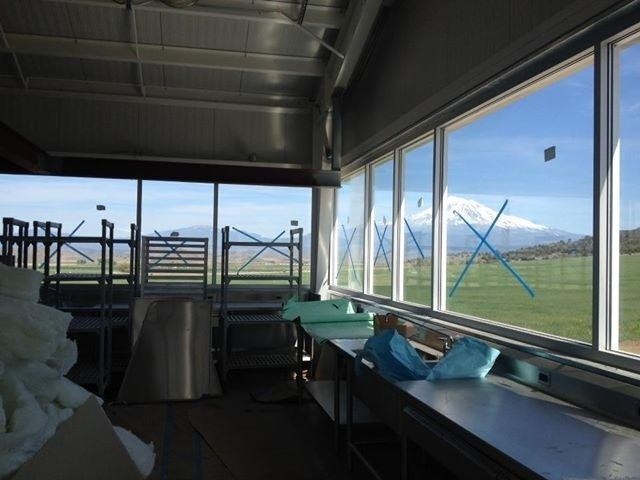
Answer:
[127,296,214,405]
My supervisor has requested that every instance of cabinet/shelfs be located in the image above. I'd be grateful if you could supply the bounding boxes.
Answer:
[218,226,304,385]
[0,213,144,408]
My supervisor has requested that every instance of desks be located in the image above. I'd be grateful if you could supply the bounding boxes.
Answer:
[305,323,394,461]
[398,373,640,480]
